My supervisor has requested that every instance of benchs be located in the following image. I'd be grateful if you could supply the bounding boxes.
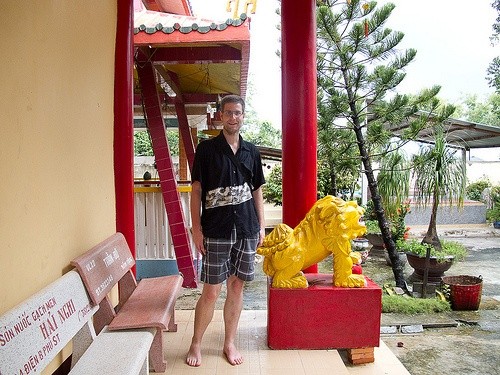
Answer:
[0,268,159,375]
[71,232,184,372]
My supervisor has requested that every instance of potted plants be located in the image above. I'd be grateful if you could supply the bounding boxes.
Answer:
[361,122,469,282]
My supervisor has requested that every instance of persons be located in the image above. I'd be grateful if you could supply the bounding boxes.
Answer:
[187,94,265,367]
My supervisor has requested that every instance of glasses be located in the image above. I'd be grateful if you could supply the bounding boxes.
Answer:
[221,110,243,118]
